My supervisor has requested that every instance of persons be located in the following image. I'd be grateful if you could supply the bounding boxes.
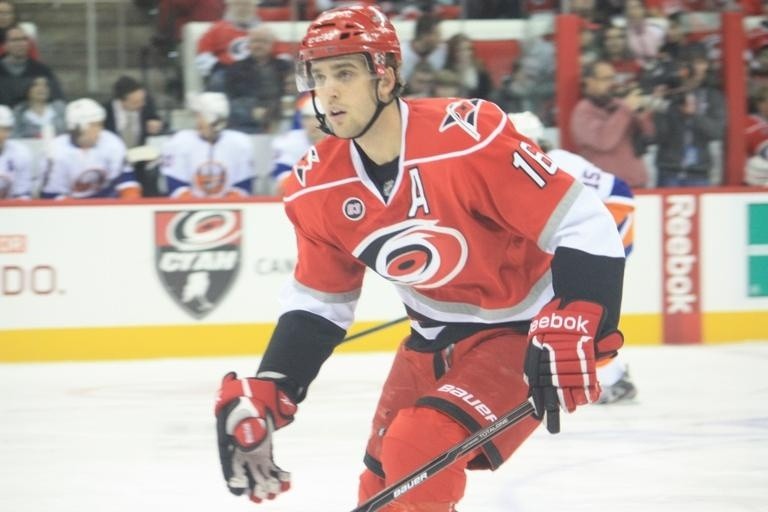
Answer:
[508,111,638,403]
[215,4,628,511]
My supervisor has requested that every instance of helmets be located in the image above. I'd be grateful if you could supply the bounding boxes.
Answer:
[293,3,404,142]
[187,89,231,131]
[66,96,109,131]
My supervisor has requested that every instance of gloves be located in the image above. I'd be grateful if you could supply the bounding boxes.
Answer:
[520,293,625,436]
[213,369,298,505]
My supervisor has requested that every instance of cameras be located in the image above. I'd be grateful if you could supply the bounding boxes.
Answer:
[614,88,671,112]
